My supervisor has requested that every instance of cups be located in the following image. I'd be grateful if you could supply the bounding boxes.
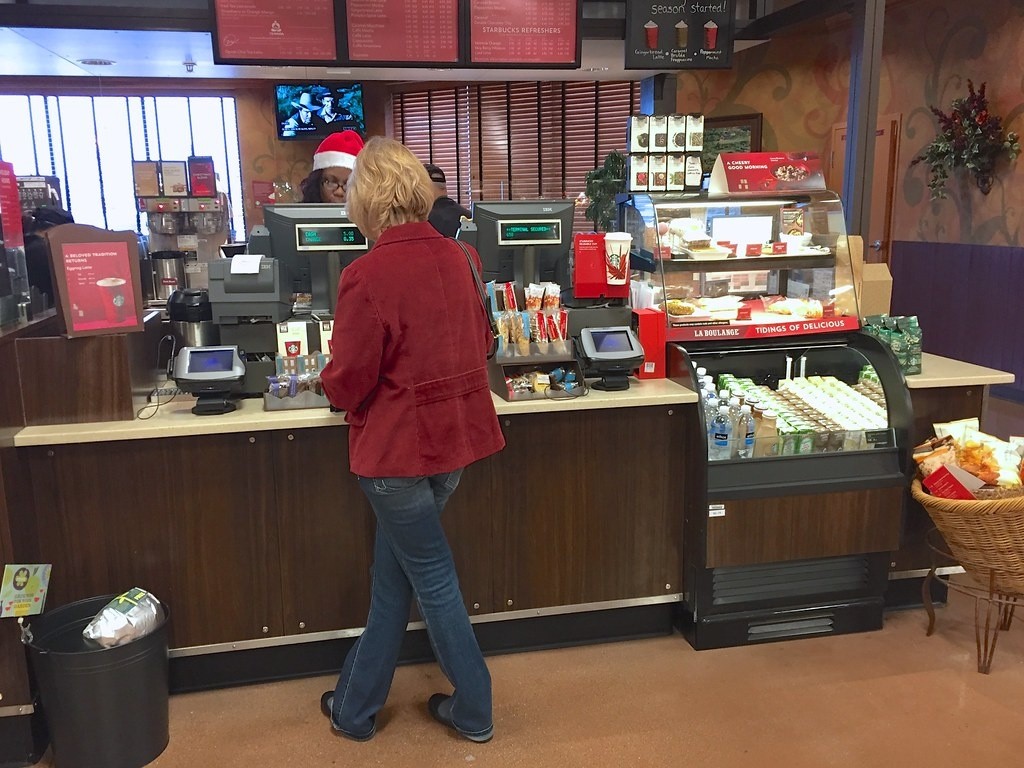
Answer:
[604,231,633,286]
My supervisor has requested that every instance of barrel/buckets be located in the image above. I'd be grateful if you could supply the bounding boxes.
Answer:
[20,593,171,768]
[166,287,219,355]
[152,250,188,300]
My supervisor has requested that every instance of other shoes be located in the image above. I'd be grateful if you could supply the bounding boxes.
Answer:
[429,692,494,743]
[321,691,336,717]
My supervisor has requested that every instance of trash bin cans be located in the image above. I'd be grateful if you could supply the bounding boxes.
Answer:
[26,593,169,768]
[166,288,221,356]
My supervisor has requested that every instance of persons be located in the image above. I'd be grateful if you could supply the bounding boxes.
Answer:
[0,205,75,299]
[319,133,511,745]
[286,82,359,138]
[297,129,364,203]
[423,162,472,237]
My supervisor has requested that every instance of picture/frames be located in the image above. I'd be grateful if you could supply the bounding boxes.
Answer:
[703,113,762,177]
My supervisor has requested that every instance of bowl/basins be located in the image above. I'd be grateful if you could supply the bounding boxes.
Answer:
[778,231,813,251]
[219,243,247,258]
[769,161,811,189]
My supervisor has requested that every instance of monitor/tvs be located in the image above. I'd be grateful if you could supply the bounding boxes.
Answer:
[273,82,368,142]
[472,198,576,286]
[263,202,374,293]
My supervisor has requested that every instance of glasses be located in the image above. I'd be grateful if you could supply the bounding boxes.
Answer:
[320,179,346,192]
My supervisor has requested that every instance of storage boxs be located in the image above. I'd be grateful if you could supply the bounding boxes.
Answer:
[631,308,666,379]
[861,261,893,326]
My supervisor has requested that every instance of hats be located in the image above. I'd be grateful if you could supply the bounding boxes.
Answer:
[312,132,364,172]
[291,93,321,111]
[32,205,75,224]
[423,163,446,183]
[316,88,345,101]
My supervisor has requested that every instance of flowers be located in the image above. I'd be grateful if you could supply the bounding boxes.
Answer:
[909,78,1020,201]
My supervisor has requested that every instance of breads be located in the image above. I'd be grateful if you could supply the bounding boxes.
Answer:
[658,300,694,315]
[768,297,824,317]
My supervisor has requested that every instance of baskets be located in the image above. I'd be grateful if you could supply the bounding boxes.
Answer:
[912,478,1024,599]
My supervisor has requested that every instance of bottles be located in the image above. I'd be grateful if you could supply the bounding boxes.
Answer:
[691,360,780,461]
